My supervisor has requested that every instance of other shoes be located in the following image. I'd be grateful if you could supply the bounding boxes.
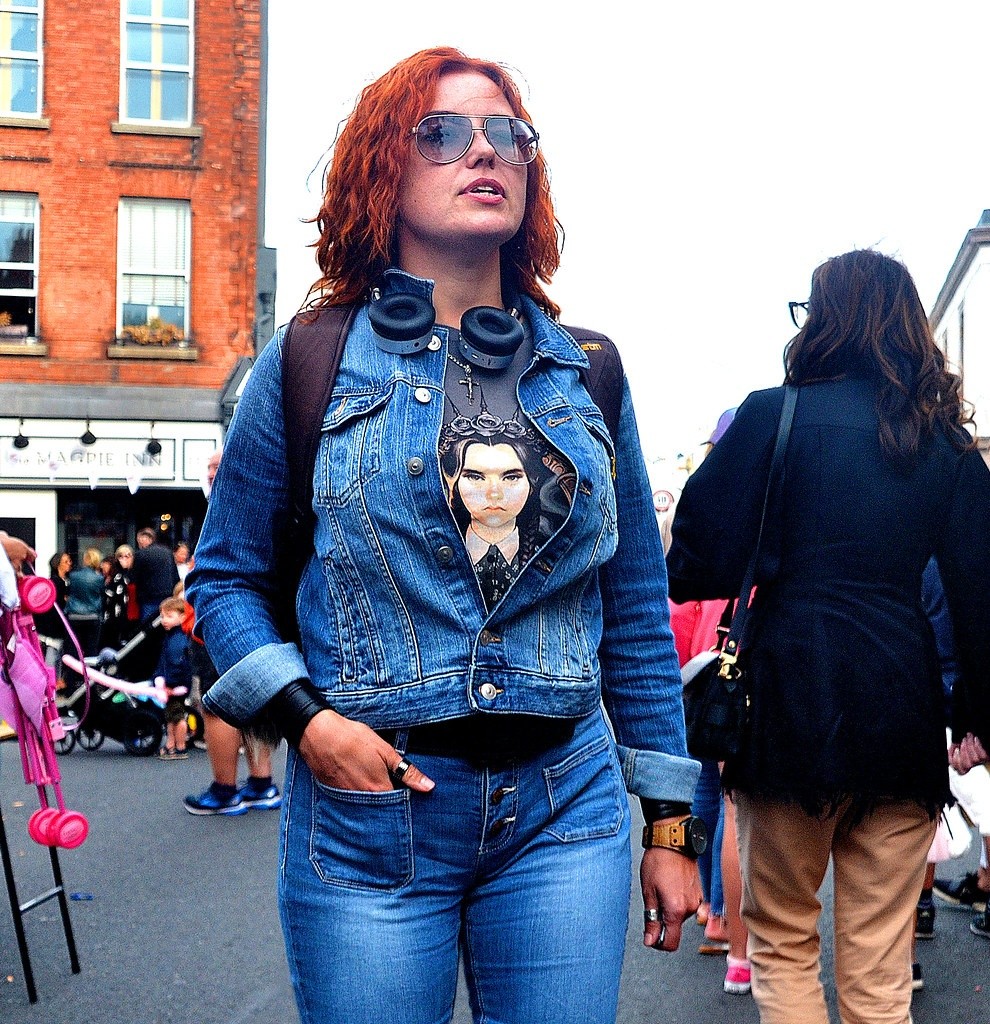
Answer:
[969,903,990,938]
[914,906,936,938]
[912,963,923,989]
[722,961,752,994]
[151,744,190,761]
[933,871,990,912]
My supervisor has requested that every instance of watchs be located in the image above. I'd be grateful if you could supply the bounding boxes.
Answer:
[641,815,708,860]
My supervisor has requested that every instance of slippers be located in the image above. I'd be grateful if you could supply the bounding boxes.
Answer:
[698,940,729,958]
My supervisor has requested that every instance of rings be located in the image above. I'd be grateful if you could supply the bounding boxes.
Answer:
[394,758,411,780]
[644,909,661,923]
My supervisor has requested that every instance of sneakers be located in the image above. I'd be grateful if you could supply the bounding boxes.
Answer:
[181,781,246,816]
[236,780,284,812]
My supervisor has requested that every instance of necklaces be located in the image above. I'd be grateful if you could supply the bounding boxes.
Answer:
[445,354,481,404]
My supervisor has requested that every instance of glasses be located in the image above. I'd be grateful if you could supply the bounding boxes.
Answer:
[788,300,813,329]
[410,114,541,166]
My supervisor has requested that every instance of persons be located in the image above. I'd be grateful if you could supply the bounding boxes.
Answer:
[647,253,990,1024]
[184,451,283,814]
[0,524,244,759]
[184,50,705,1024]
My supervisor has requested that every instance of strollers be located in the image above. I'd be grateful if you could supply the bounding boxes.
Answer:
[54,613,204,757]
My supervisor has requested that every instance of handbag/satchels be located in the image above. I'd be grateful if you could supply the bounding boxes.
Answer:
[679,647,748,760]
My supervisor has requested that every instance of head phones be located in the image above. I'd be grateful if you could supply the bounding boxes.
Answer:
[364,280,527,369]
